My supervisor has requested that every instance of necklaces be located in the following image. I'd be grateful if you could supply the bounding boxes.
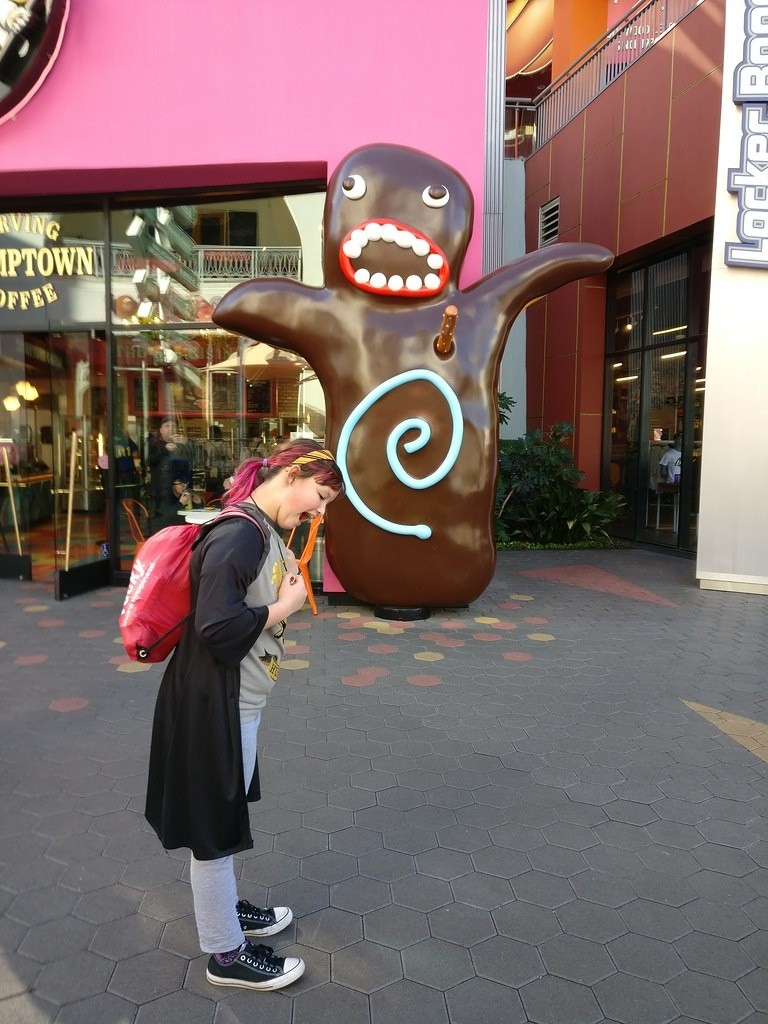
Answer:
[249,493,297,586]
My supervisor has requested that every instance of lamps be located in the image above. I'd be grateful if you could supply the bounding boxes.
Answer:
[3,381,39,411]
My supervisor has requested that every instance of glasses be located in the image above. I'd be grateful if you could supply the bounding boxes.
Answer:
[173,481,190,486]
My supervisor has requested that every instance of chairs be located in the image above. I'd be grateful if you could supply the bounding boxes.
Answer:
[123,499,149,560]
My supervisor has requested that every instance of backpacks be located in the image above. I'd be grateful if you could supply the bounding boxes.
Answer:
[119,505,266,663]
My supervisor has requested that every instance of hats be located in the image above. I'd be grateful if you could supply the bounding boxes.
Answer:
[159,412,179,427]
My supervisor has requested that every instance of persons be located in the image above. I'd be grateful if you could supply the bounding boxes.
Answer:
[659,431,682,481]
[108,415,205,538]
[206,425,234,479]
[143,437,347,992]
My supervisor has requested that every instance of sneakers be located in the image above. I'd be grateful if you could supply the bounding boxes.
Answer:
[206,941,305,993]
[226,899,292,939]
